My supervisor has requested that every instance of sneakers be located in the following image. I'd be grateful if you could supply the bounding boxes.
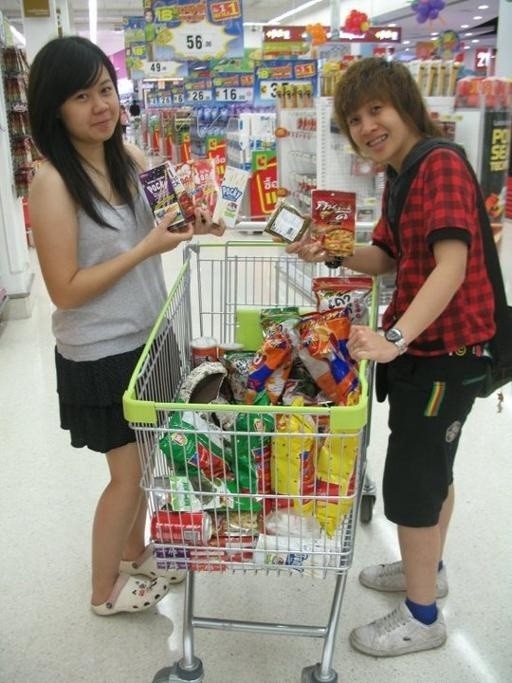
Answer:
[358,559,449,599]
[349,599,448,658]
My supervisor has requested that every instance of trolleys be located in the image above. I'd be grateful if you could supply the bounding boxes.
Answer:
[123,239,383,682]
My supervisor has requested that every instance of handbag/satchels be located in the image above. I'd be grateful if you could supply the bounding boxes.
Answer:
[475,304,512,399]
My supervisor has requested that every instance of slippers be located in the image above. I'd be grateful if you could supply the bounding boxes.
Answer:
[119,540,188,585]
[89,571,170,617]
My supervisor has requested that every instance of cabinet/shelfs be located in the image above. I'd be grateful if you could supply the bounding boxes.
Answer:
[221,113,277,234]
[275,96,482,318]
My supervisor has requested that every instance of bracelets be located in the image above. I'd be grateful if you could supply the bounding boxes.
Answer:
[324,254,343,268]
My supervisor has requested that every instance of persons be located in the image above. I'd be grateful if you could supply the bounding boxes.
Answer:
[118,104,130,139]
[273,56,503,658]
[24,35,226,615]
[129,99,141,129]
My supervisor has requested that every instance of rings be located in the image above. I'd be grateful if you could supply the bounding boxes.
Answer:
[351,343,357,349]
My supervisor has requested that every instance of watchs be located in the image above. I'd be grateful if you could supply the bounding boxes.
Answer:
[383,328,410,356]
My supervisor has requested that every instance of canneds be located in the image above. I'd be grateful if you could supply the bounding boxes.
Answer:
[150,511,212,546]
[190,337,244,369]
